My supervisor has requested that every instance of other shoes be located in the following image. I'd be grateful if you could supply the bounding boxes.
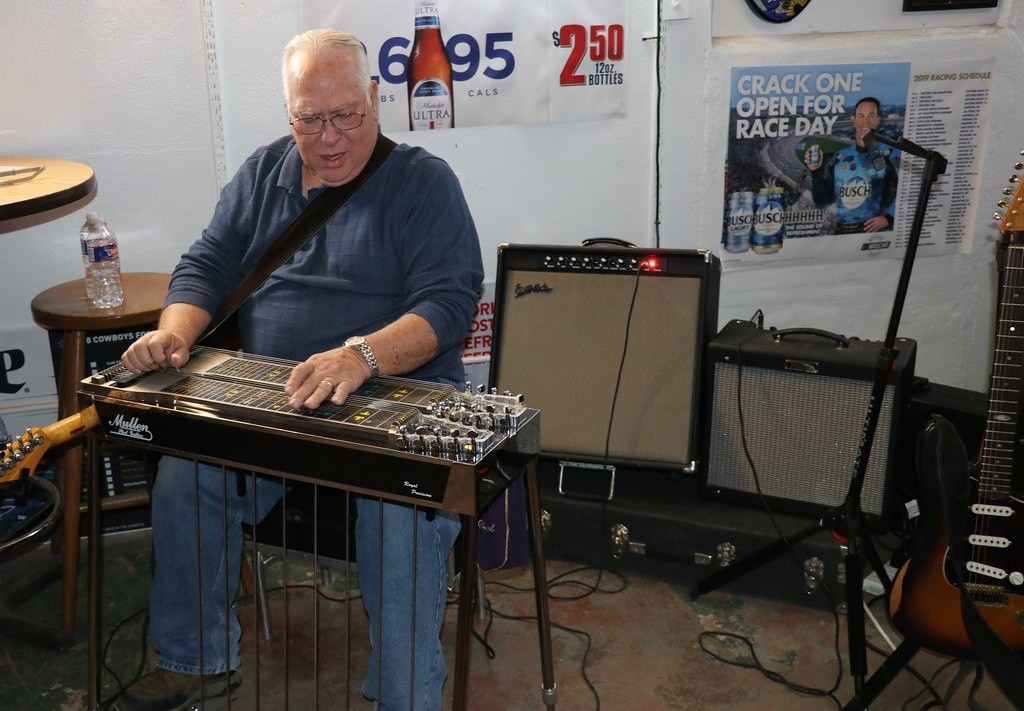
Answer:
[125,665,242,711]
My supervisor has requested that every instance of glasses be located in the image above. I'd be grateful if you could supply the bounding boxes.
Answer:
[289,97,366,135]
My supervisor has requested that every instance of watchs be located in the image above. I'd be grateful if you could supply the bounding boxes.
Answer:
[344,336,382,378]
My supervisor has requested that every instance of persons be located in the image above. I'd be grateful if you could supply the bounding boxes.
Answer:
[804,96,898,236]
[113,29,486,711]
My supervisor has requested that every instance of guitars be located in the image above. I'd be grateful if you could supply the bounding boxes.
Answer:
[0,402,101,486]
[883,148,1024,660]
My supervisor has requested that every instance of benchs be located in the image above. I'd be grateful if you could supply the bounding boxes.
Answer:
[242,511,487,641]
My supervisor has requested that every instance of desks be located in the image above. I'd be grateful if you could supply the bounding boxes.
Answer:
[0,159,96,560]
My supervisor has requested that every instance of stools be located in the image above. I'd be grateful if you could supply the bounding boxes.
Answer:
[31,274,196,636]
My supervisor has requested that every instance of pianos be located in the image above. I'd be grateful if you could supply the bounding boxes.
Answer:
[70,342,559,711]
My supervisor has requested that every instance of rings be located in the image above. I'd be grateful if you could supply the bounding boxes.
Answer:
[320,380,335,390]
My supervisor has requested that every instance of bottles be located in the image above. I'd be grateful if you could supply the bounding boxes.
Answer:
[79,211,123,312]
[406,0,456,132]
